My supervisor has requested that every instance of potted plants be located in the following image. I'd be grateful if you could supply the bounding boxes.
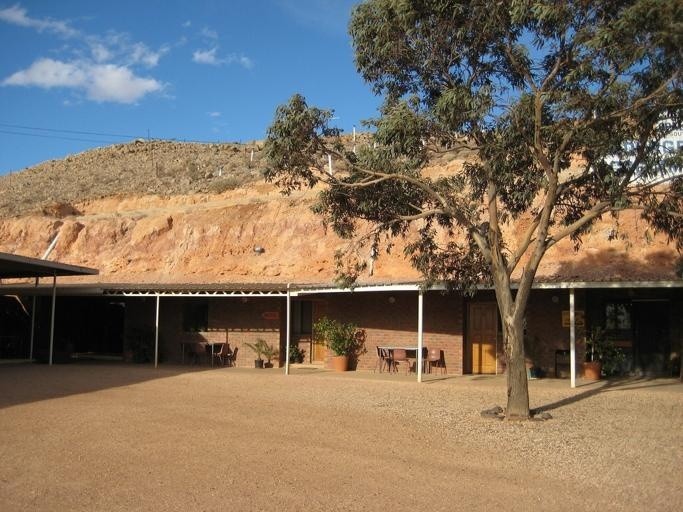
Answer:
[523,331,547,378]
[313,316,355,371]
[576,325,619,380]
[285,345,298,363]
[246,341,264,368]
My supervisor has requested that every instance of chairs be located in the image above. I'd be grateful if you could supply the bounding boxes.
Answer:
[423,347,442,375]
[212,343,228,367]
[555,350,570,379]
[374,346,393,373]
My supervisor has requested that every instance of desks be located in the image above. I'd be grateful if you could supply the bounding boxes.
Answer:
[182,342,210,367]
[392,346,418,374]
